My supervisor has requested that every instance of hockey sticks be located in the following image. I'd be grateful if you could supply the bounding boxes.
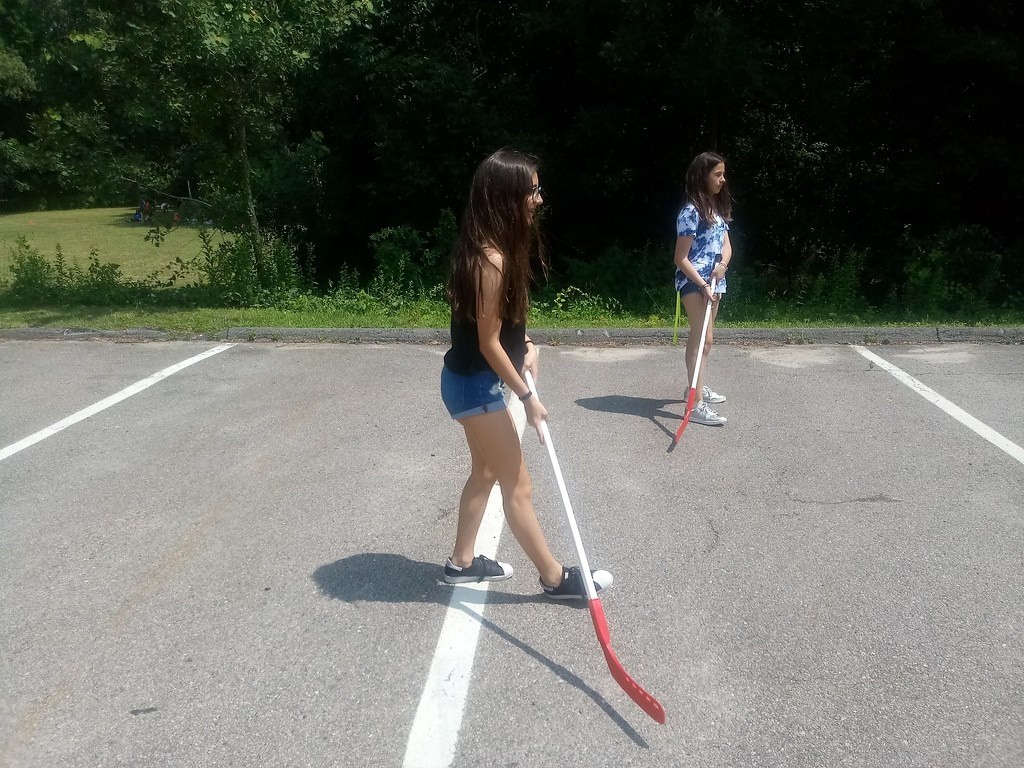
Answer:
[524,366,666,726]
[676,250,725,442]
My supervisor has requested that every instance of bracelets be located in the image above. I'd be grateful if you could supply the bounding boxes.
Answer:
[525,341,534,344]
[519,391,533,401]
[700,284,709,293]
[720,263,728,270]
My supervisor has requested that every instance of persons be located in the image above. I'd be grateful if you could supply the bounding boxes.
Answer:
[674,152,732,425]
[138,197,182,222]
[440,150,613,599]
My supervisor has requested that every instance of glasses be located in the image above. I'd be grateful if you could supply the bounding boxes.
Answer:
[531,187,541,200]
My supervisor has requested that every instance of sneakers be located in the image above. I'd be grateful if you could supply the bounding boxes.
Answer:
[684,401,727,425]
[539,565,614,600]
[444,555,513,584]
[683,386,726,403]
